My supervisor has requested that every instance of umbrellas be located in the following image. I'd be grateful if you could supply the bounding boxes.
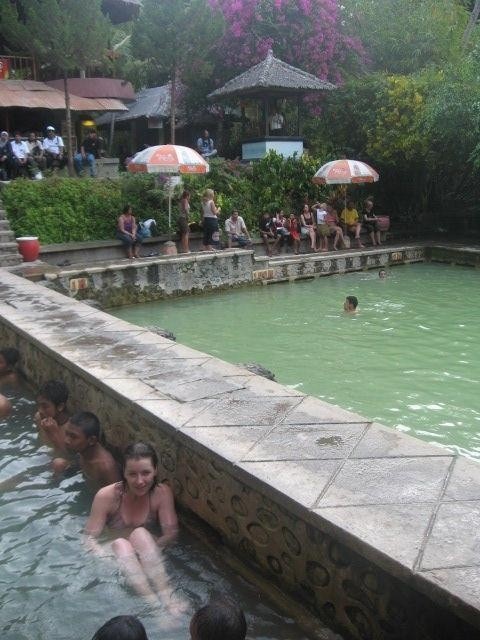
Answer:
[311,159,380,235]
[128,143,209,228]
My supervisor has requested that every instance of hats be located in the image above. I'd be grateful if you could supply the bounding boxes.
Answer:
[46,126,55,131]
[2,131,8,134]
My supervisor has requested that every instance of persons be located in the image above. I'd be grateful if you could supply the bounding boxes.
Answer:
[196,129,217,161]
[0,347,121,483]
[269,111,284,135]
[116,205,144,258]
[224,210,251,248]
[200,189,221,250]
[177,190,191,253]
[189,603,247,640]
[92,615,148,640]
[0,123,65,179]
[74,128,97,178]
[259,200,383,256]
[344,296,358,311]
[379,270,386,278]
[83,442,188,630]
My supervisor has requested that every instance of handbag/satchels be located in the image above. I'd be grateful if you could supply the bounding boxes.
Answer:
[138,222,161,237]
